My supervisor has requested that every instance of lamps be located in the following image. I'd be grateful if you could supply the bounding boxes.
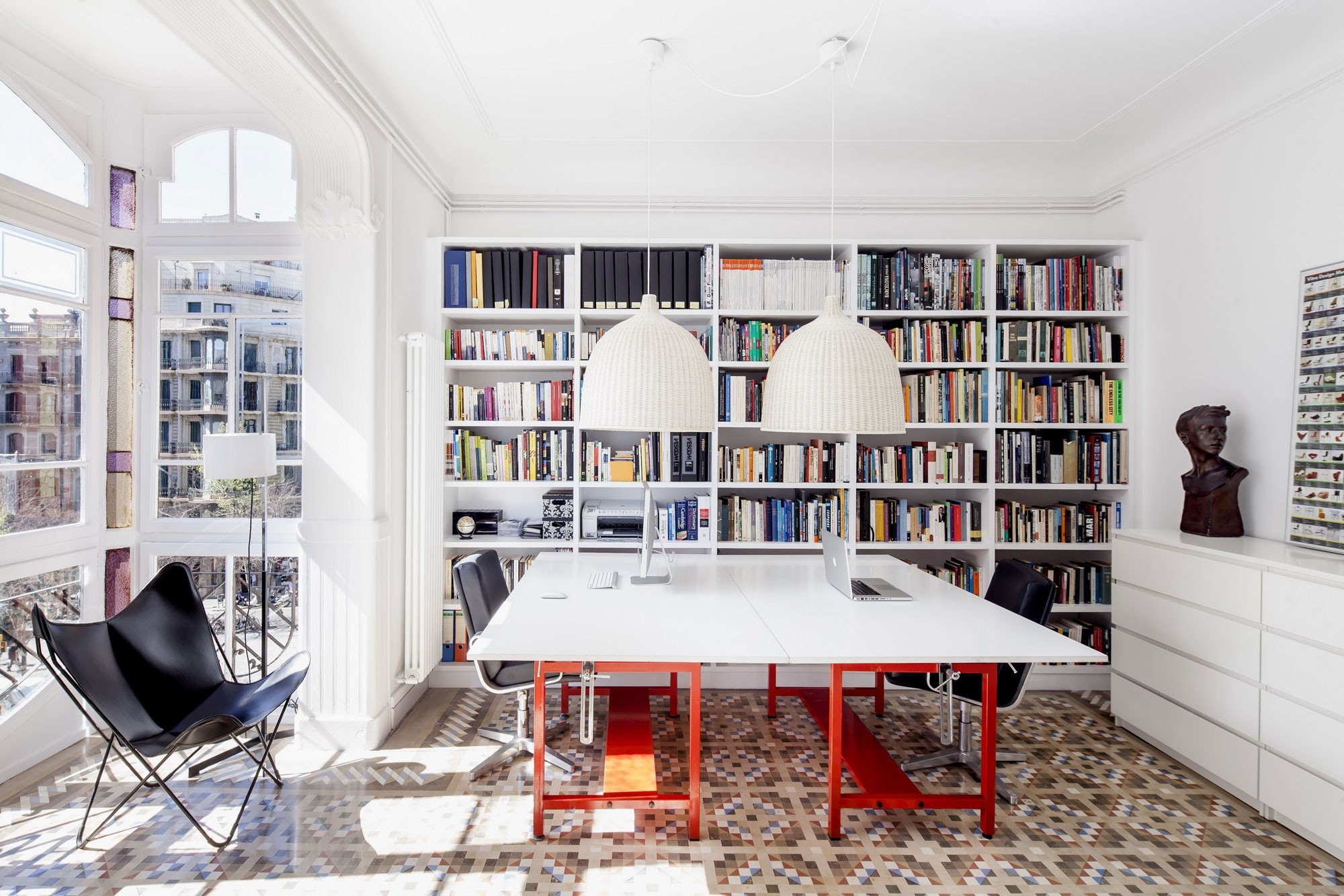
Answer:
[188,427,298,780]
[579,36,720,432]
[763,35,913,437]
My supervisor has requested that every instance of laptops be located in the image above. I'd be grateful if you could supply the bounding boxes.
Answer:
[821,529,913,602]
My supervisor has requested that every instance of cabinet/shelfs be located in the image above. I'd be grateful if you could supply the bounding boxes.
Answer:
[417,228,1126,704]
[1104,525,1344,868]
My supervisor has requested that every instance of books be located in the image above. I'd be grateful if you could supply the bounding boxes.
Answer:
[442,244,1130,668]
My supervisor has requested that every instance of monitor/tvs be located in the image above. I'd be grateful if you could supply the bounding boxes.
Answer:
[631,481,675,587]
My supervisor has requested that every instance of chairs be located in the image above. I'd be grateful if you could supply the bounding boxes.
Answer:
[34,561,312,846]
[884,558,1054,808]
[453,548,596,782]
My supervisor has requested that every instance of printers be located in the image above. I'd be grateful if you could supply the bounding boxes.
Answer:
[582,498,658,541]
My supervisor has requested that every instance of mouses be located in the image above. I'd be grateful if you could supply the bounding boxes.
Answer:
[540,591,567,598]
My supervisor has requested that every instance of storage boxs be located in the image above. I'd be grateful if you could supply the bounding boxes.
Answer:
[446,243,712,311]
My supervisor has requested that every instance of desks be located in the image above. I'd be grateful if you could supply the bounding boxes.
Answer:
[715,556,1111,842]
[467,549,788,846]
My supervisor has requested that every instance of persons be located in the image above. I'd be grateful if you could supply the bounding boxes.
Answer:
[1174,404,1251,538]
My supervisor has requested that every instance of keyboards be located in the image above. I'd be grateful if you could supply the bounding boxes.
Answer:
[587,571,618,590]
